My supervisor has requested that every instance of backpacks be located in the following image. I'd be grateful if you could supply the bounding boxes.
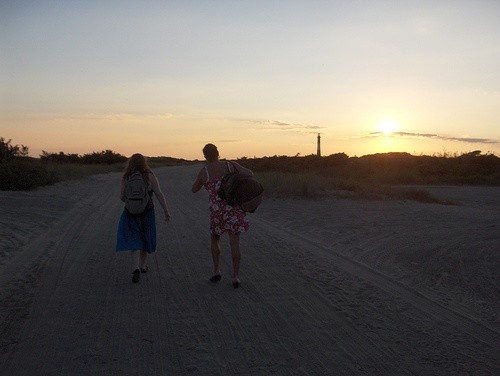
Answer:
[124,164,152,216]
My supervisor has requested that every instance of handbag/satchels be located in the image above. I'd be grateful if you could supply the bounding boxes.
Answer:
[217,173,264,214]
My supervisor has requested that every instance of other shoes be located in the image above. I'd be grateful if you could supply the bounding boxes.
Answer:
[141,265,148,274]
[232,278,241,288]
[132,269,140,283]
[209,275,221,282]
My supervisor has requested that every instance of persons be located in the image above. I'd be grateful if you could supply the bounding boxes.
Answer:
[116,152,173,285]
[192,142,257,291]
